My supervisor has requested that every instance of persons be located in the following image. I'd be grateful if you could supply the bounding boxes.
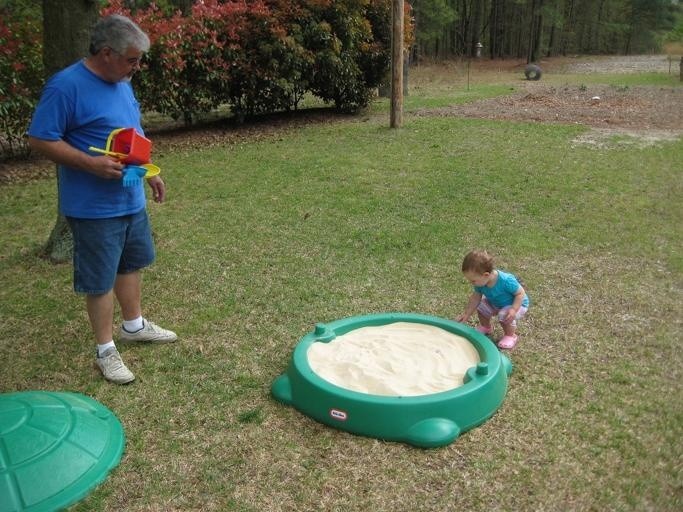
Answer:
[453,250,529,348]
[28,14,178,385]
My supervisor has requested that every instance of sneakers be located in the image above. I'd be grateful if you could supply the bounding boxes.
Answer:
[120,318,178,343]
[497,332,520,349]
[94,346,136,385]
[473,323,495,335]
